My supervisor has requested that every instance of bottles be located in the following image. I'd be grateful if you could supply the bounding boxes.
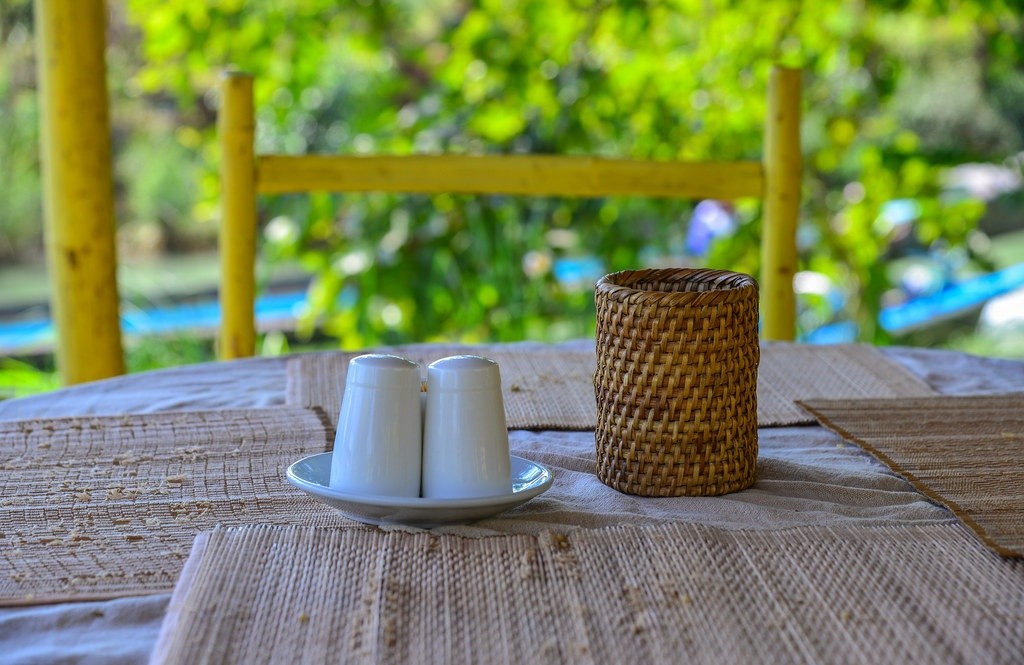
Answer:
[422,354,512,500]
[328,355,425,501]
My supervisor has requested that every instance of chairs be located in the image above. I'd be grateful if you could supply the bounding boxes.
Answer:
[222,59,806,362]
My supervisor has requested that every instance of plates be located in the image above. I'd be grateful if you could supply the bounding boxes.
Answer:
[285,448,556,527]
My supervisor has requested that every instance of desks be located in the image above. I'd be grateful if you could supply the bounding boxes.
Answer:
[0,335,1024,665]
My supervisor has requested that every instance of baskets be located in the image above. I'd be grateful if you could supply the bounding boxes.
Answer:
[593,267,762,498]
